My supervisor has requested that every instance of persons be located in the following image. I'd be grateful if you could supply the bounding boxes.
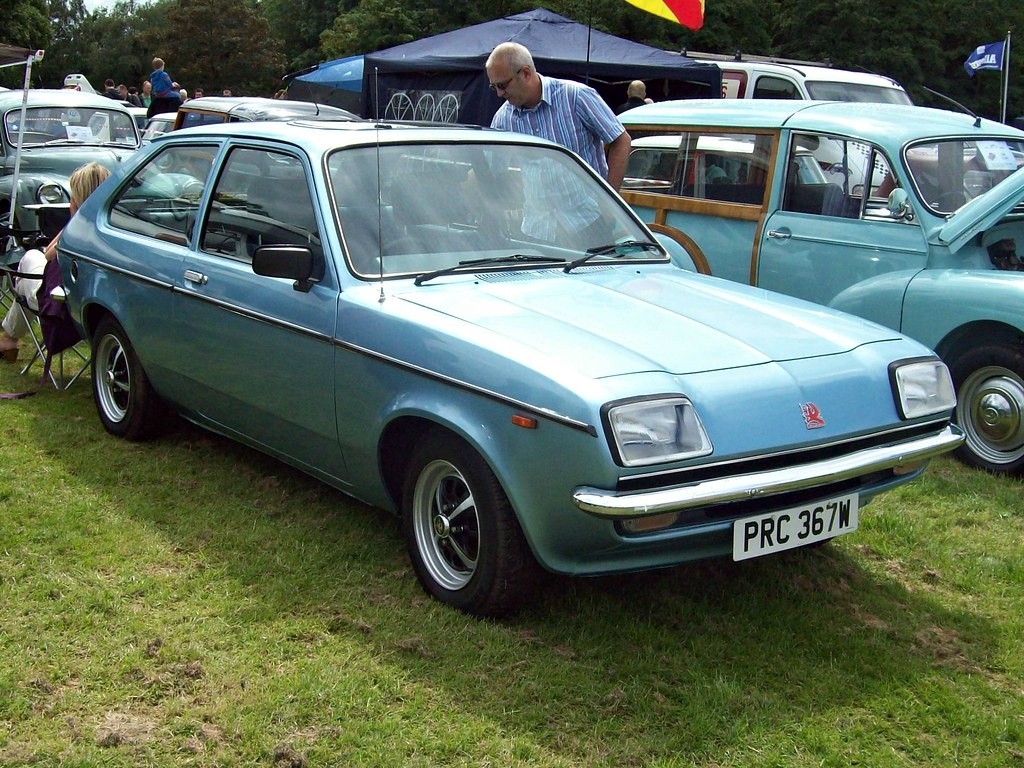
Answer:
[0,160,114,362]
[104,52,286,121]
[460,42,632,252]
[613,80,728,194]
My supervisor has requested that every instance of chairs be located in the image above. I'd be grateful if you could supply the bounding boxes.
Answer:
[390,171,476,253]
[246,175,319,235]
[0,226,91,389]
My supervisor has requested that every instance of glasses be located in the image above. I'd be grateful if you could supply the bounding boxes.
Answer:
[488,65,531,92]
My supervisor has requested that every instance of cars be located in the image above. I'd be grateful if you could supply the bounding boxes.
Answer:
[49,119,968,620]
[604,99,1024,480]
[1,88,363,258]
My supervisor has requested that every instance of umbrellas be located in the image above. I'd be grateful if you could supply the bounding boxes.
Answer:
[585,0,704,87]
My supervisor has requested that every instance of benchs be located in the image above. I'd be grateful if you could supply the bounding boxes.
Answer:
[691,183,842,219]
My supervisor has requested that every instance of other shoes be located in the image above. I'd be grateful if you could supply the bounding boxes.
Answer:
[0,349,19,363]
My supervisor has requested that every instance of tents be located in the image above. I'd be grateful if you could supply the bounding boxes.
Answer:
[280,6,722,133]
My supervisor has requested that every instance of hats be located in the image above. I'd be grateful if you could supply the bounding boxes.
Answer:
[152,58,165,69]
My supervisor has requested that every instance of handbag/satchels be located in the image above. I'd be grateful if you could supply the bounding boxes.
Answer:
[37,252,84,356]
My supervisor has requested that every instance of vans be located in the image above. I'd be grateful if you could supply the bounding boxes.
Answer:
[663,49,925,201]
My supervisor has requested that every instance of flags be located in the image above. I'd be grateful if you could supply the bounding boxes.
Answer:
[963,38,1006,79]
